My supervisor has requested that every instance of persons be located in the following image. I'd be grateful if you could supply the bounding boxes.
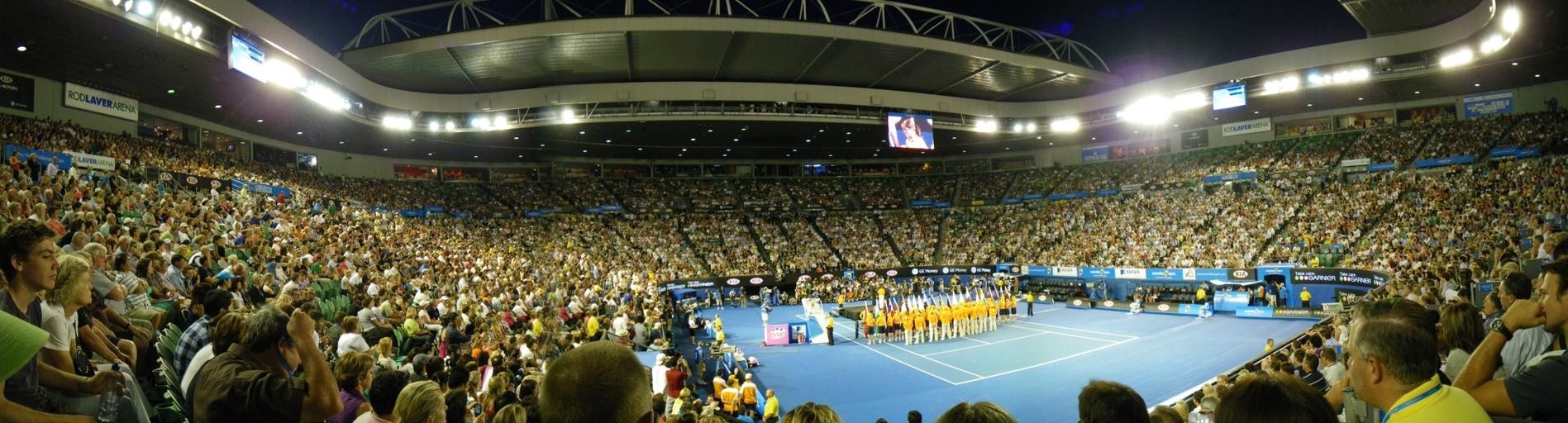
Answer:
[1077,379,1149,423]
[780,100,1568,317]
[852,316,1018,346]
[779,401,844,423]
[1148,316,1568,423]
[824,312,834,346]
[907,409,922,423]
[0,105,781,423]
[935,401,1018,423]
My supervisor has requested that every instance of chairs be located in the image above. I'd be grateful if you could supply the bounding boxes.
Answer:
[1320,248,1339,266]
[46,209,409,423]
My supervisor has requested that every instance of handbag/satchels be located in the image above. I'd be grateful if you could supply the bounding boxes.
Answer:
[74,349,95,377]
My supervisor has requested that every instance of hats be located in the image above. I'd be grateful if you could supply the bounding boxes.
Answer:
[217,271,235,279]
[145,251,164,260]
[0,309,50,383]
[124,218,134,224]
[149,228,162,236]
[656,353,666,365]
[200,245,212,252]
[419,300,431,306]
